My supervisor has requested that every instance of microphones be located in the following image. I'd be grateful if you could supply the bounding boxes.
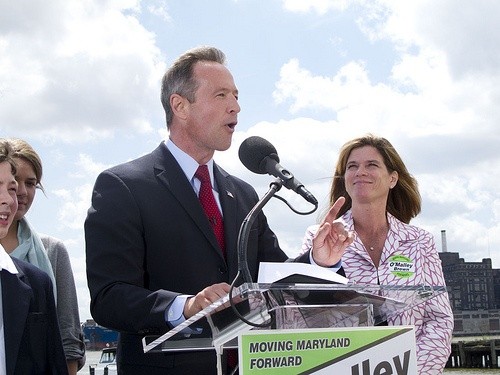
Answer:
[238,135,318,205]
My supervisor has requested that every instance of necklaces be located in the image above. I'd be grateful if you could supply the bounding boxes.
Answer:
[354,220,388,251]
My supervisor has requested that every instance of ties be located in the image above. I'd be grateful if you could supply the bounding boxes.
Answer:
[195,165,225,260]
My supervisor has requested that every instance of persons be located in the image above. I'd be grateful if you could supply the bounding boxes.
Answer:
[84,47,355,375]
[0,140,86,375]
[0,138,68,375]
[297,137,454,375]
